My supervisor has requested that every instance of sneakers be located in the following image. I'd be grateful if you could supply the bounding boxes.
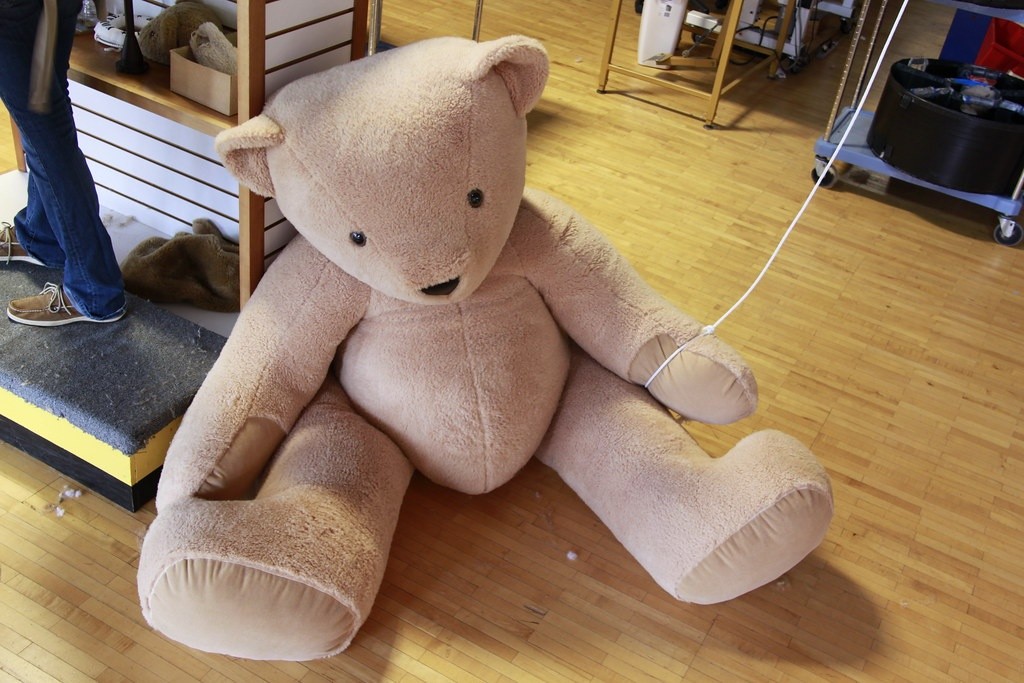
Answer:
[0,222,46,266]
[6,281,126,326]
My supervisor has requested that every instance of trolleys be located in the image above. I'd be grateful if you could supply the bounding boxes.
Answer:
[812,3,1024,250]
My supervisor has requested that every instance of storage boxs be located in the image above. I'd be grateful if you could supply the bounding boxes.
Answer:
[169,31,238,117]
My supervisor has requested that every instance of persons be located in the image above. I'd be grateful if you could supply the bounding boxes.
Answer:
[0,0,130,328]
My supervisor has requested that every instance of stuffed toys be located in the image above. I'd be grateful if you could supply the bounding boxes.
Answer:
[133,34,832,662]
[136,0,221,64]
